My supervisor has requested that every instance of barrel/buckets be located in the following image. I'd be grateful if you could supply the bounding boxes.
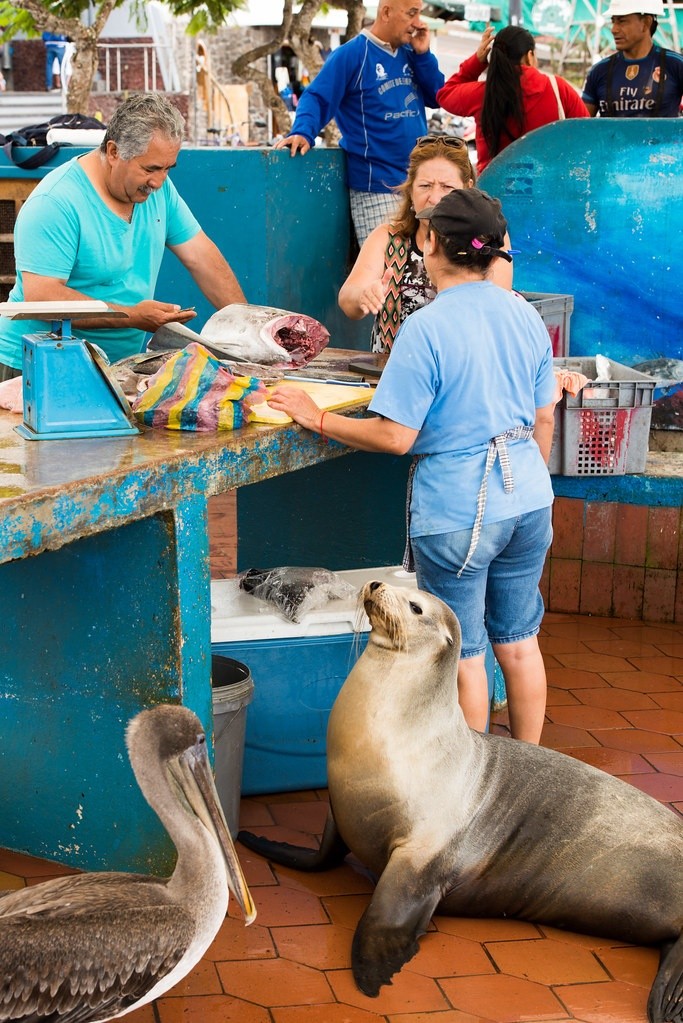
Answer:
[212,654,254,846]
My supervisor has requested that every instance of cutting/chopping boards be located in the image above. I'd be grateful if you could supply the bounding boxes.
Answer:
[251,381,377,424]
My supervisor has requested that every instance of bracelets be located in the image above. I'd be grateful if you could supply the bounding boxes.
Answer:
[320,411,328,443]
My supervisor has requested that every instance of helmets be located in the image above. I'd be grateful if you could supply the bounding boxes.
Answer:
[602,0,664,17]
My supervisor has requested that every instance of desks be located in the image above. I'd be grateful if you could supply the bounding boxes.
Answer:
[0,346,406,876]
[0,144,378,350]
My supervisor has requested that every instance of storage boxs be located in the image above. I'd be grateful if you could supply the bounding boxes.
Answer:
[209,564,420,798]
[549,355,659,476]
[519,291,574,358]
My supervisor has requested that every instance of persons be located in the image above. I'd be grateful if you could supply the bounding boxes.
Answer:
[338,134,514,354]
[280,82,294,112]
[436,21,592,177]
[265,188,556,745]
[581,0,683,118]
[273,0,446,247]
[0,92,247,382]
[42,31,76,92]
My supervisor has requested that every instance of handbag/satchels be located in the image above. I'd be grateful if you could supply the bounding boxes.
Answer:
[2,113,107,170]
[132,342,268,431]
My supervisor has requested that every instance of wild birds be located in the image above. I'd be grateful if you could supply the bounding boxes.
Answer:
[0,704,258,1023]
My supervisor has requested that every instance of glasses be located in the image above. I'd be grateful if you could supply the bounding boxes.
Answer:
[417,135,468,149]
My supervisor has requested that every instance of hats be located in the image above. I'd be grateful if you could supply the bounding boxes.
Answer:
[414,188,512,262]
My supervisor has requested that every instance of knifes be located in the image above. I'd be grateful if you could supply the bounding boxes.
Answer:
[285,374,370,388]
